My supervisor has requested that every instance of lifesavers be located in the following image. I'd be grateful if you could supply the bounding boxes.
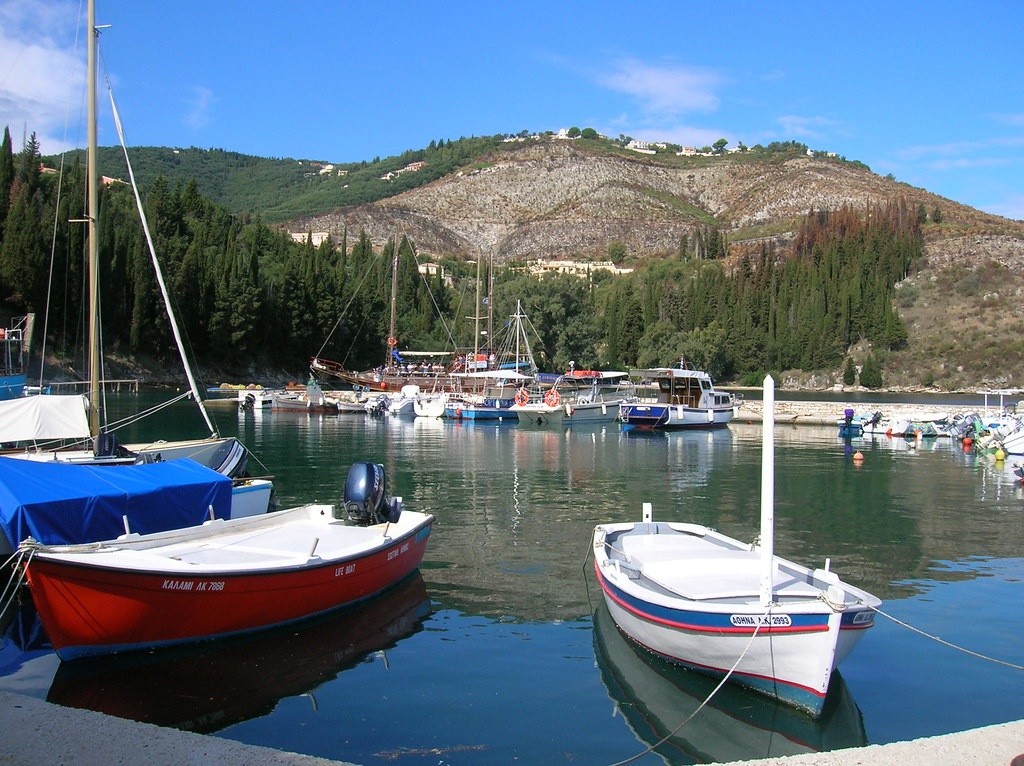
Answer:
[514,389,529,405]
[388,337,398,346]
[545,389,561,406]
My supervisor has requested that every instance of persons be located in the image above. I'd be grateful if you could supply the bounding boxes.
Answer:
[373,360,433,378]
[844,438,852,456]
[841,408,854,431]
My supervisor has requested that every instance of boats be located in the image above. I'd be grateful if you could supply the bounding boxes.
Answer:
[618,353,745,426]
[273,393,336,414]
[594,373,882,719]
[19,457,440,666]
[838,401,1024,486]
[588,597,870,766]
[236,388,299,411]
[45,570,433,738]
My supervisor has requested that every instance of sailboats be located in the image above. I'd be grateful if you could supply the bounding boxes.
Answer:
[1,0,277,581]
[309,214,629,421]
